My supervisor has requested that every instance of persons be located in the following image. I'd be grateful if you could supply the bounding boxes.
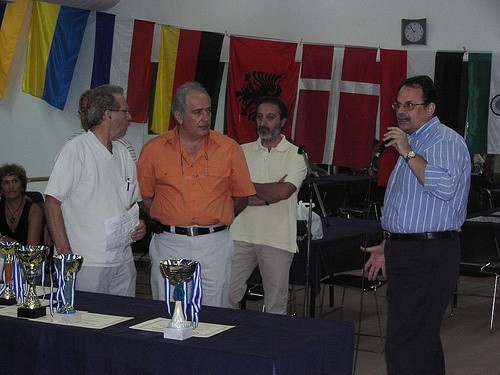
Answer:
[136,83,258,307]
[221,98,308,313]
[361,74,472,375]
[371,139,381,163]
[0,164,42,246]
[44,85,143,294]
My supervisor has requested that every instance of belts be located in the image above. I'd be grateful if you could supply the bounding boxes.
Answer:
[162,222,226,237]
[383,230,460,240]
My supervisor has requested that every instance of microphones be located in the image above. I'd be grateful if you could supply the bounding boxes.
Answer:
[374,125,399,159]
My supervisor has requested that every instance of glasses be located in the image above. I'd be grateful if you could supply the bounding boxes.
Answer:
[104,105,131,117]
[391,100,429,112]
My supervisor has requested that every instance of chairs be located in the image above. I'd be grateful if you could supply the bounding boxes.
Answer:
[239,155,500,353]
[26,190,54,270]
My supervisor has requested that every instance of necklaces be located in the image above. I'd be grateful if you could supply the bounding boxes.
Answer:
[6,200,24,223]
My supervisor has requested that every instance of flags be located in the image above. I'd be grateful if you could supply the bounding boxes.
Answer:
[377,50,500,186]
[0,0,90,111]
[90,13,379,169]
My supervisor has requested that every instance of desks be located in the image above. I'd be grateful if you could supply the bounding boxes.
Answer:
[0,288,354,375]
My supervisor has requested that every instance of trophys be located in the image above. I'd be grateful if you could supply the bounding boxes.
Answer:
[53,253,84,314]
[15,244,50,318]
[159,259,202,340]
[0,240,21,306]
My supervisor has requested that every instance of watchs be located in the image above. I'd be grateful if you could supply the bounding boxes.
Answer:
[405,151,417,163]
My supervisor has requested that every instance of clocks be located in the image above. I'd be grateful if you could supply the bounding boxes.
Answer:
[400,17,426,45]
